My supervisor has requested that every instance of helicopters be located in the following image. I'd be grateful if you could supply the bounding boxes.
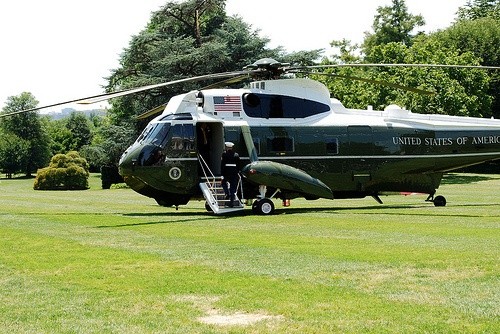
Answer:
[2,58,499,216]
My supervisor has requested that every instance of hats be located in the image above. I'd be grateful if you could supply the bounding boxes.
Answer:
[225,142,235,148]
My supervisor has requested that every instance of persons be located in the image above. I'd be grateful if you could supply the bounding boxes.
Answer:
[220,141,243,208]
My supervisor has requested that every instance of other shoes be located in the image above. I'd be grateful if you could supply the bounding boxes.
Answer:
[225,194,229,199]
